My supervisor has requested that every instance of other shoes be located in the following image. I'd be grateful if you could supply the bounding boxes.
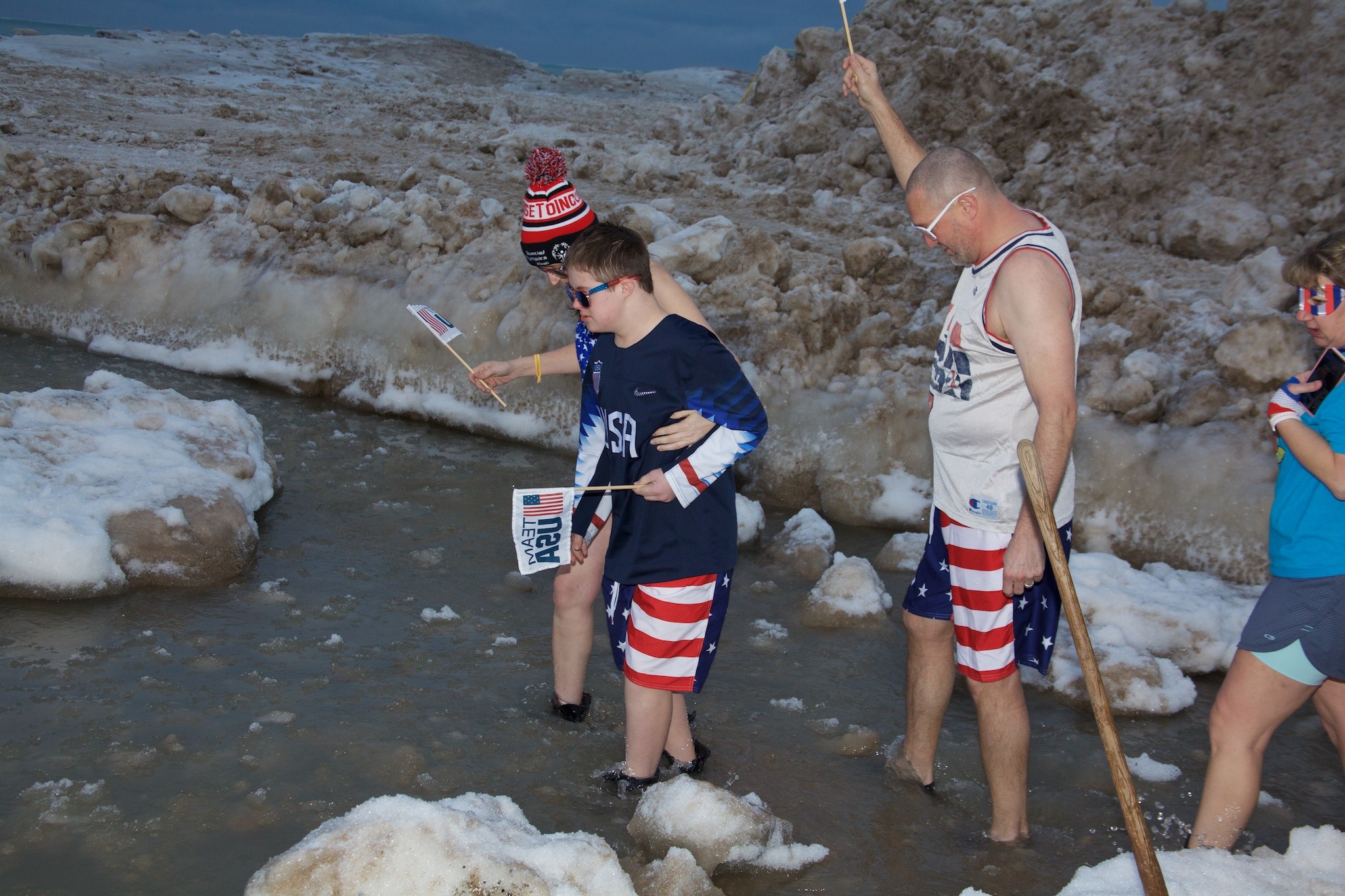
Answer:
[664,739,710,774]
[553,690,591,724]
[605,766,659,792]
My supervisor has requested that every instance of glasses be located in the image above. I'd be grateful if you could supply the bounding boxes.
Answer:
[566,272,643,309]
[910,187,977,242]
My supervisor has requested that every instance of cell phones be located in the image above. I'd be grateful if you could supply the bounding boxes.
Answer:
[1297,346,1345,416]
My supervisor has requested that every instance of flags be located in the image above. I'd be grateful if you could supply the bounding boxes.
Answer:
[412,305,467,343]
[512,489,574,576]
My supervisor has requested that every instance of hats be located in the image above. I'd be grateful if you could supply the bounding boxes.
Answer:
[521,146,599,266]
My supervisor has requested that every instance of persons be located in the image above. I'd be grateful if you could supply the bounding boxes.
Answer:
[842,54,1081,840]
[468,146,741,722]
[1184,230,1345,849]
[561,221,767,793]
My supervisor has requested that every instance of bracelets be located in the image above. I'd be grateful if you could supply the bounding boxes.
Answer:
[534,354,541,383]
